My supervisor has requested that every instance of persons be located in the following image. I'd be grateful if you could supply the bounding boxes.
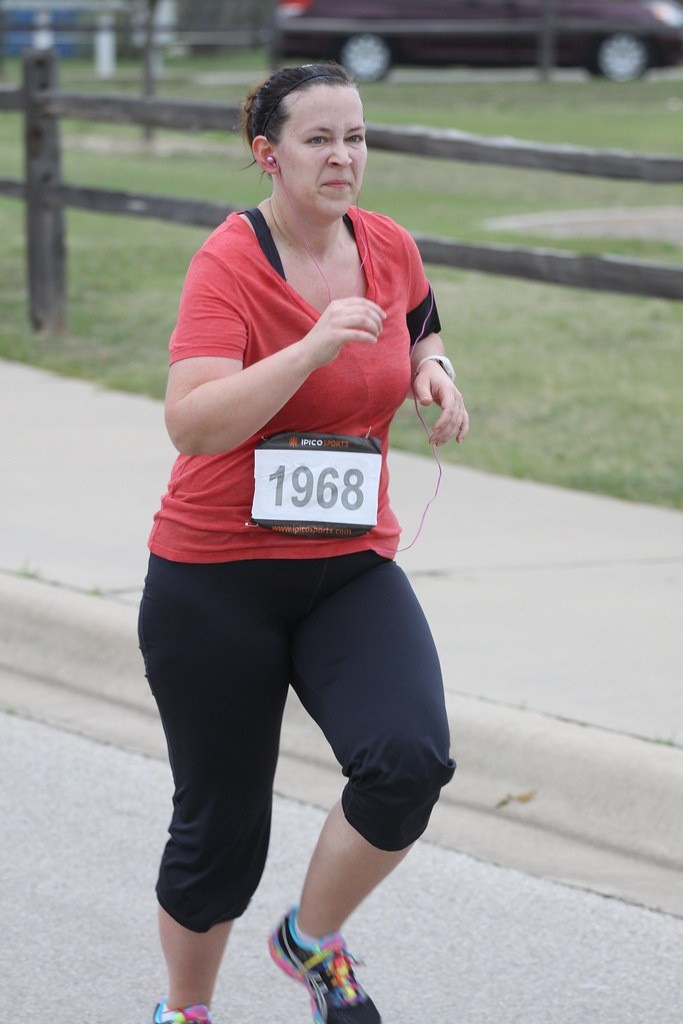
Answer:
[133,61,476,1022]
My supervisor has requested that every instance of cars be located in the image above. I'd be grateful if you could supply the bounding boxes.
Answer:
[267,2,683,86]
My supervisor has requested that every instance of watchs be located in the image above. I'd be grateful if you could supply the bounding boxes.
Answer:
[415,354,457,382]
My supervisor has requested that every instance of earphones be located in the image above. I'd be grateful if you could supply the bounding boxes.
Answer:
[267,156,277,169]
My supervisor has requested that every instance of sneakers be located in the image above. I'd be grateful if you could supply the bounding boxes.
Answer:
[151,999,210,1024]
[267,907,382,1024]
